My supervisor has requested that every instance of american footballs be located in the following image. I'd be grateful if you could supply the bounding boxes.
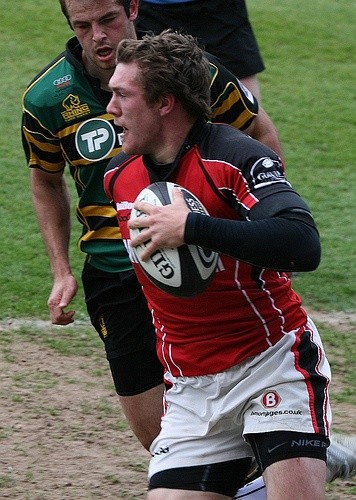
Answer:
[129,182,219,297]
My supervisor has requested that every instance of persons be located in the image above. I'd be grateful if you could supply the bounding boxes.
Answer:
[20,1,283,489]
[131,1,271,113]
[104,23,354,498]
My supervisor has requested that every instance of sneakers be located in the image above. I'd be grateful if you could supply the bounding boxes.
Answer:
[325,429,356,483]
